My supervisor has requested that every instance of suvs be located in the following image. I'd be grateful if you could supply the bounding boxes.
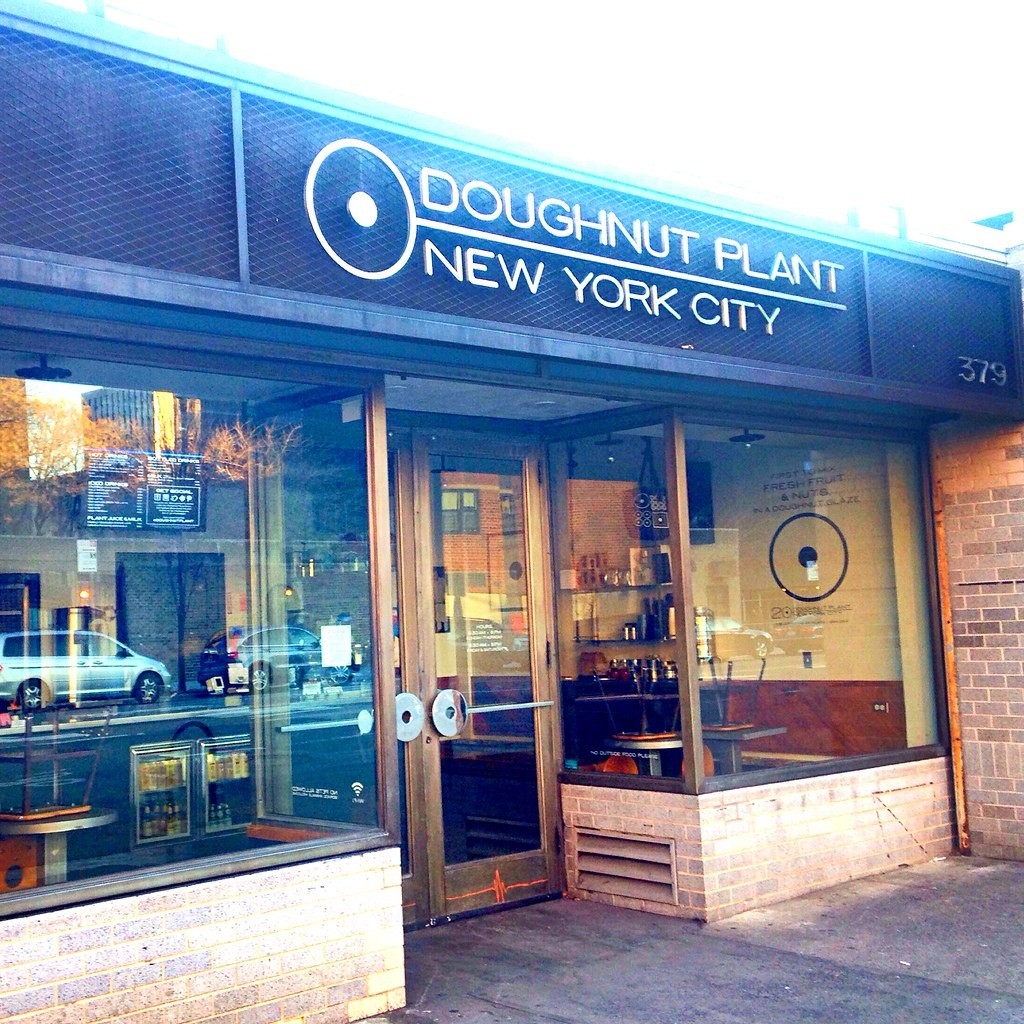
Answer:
[464,618,532,674]
[198,623,362,696]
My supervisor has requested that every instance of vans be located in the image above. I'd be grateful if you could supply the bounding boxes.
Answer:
[0,630,173,709]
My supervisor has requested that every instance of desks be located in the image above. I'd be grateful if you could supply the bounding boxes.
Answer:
[614,726,788,776]
[0,807,118,886]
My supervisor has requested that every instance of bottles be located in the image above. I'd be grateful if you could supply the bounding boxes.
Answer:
[138,756,182,790]
[610,658,676,682]
[632,627,636,639]
[210,803,231,827]
[624,628,629,640]
[207,750,249,782]
[142,801,181,836]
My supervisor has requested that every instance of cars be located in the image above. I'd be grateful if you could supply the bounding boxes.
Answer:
[775,613,856,654]
[695,617,774,665]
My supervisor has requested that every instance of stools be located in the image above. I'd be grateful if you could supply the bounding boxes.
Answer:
[0,710,112,820]
[591,657,766,740]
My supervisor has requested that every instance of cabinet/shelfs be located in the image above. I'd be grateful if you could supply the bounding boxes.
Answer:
[571,585,676,646]
